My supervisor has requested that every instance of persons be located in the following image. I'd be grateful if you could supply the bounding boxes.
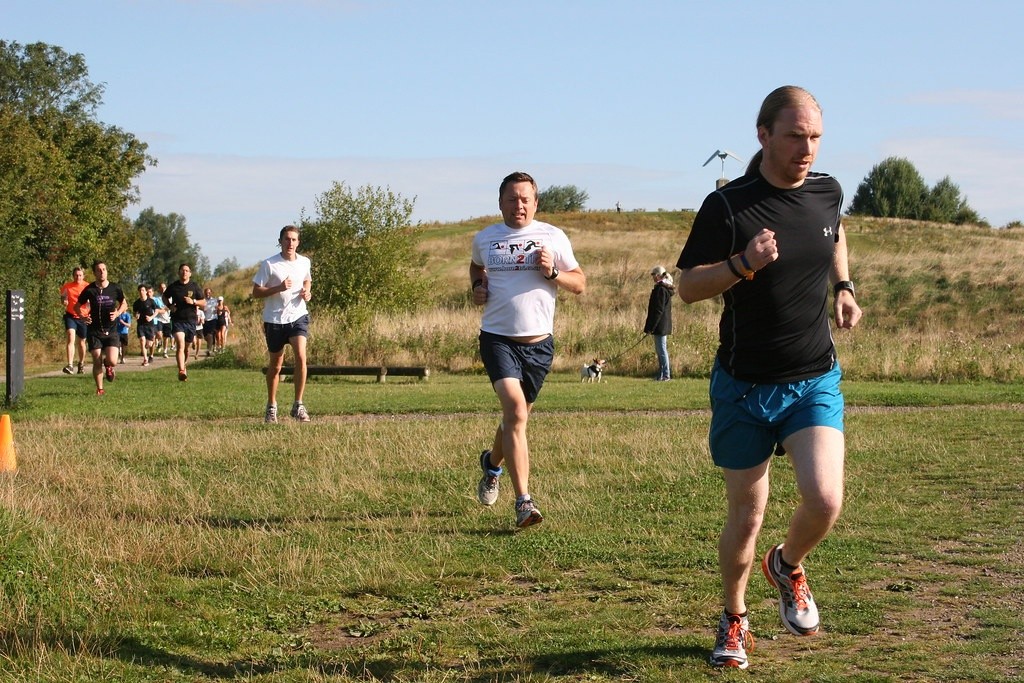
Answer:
[192,306,205,359]
[676,85,863,674]
[75,261,127,395]
[204,288,230,354]
[162,263,207,381]
[133,285,158,366]
[148,283,176,358]
[616,202,621,213]
[643,267,675,381]
[60,268,90,374]
[252,225,312,424]
[470,172,586,526]
[117,304,131,365]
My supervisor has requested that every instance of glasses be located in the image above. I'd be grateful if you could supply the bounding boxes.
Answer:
[651,273,656,276]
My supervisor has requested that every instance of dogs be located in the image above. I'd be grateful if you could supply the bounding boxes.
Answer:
[580,358,606,383]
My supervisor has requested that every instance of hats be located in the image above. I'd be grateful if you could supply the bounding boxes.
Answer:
[217,297,223,301]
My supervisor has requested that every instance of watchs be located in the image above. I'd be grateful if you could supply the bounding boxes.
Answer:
[833,281,855,298]
[545,268,558,280]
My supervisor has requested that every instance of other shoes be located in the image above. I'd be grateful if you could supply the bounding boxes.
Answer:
[654,377,670,382]
[118,344,227,366]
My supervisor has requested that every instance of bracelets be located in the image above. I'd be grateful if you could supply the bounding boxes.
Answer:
[740,251,755,274]
[472,279,482,290]
[727,257,744,279]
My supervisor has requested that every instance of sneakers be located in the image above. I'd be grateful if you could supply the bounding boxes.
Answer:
[265,401,278,424]
[77,363,85,374]
[708,604,754,669]
[515,497,542,527]
[103,359,115,382]
[763,541,820,636]
[97,389,104,396]
[478,450,502,506]
[290,402,310,421]
[63,366,73,375]
[179,362,188,382]
